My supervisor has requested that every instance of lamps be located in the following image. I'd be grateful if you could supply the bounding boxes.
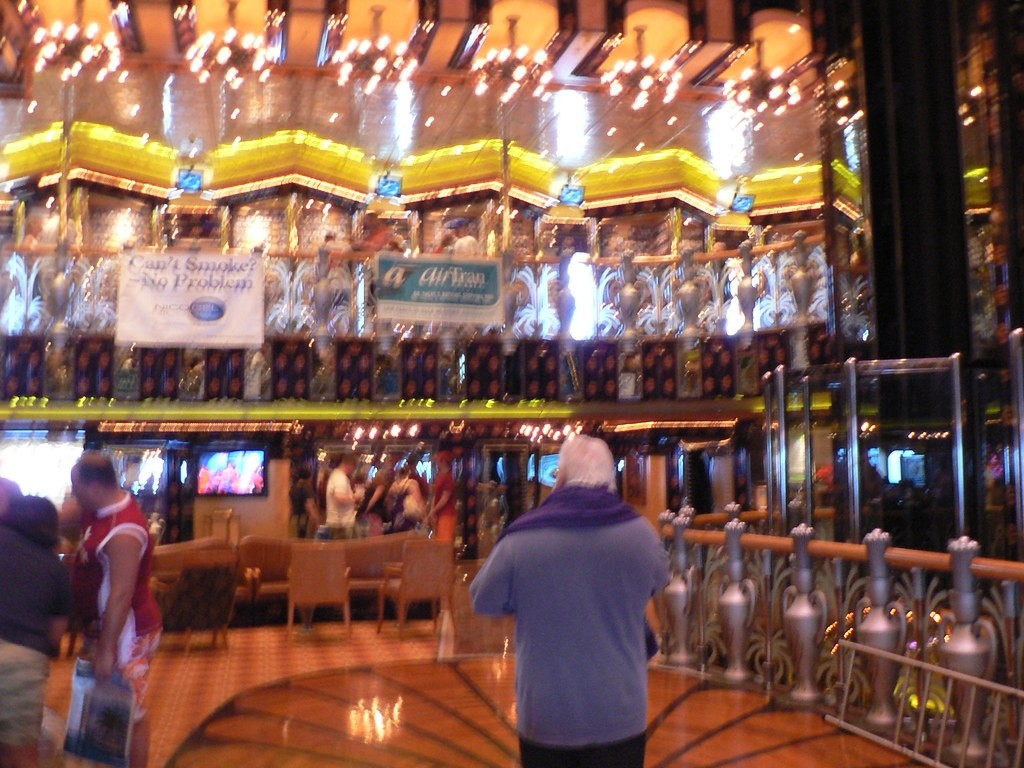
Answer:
[331,7,417,94]
[600,27,680,112]
[184,0,270,91]
[470,16,554,102]
[29,1,128,85]
[722,39,799,116]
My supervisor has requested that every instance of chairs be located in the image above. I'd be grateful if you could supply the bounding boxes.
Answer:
[148,552,241,653]
[375,539,454,641]
[284,541,353,639]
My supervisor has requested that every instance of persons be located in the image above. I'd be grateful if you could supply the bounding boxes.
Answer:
[468,431,671,768]
[298,449,457,554]
[1,449,161,767]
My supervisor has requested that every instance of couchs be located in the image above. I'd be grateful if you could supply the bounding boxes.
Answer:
[238,531,442,627]
[151,538,254,628]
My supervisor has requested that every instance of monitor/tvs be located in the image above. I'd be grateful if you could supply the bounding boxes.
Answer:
[194,449,267,497]
